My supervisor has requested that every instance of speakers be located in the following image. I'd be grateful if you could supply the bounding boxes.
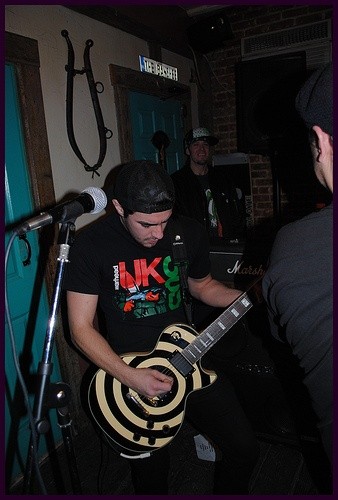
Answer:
[210,155,255,247]
[188,12,233,48]
[235,53,308,158]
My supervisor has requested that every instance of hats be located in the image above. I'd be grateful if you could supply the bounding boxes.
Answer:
[184,128,220,146]
[294,63,333,135]
[109,159,174,212]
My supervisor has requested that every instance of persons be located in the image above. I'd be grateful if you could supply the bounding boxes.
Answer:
[258,58,334,458]
[64,162,244,499]
[171,126,249,246]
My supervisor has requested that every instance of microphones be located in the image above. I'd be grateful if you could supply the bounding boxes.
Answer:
[18,187,108,234]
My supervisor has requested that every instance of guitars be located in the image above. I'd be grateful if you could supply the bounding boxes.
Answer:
[87,277,271,461]
